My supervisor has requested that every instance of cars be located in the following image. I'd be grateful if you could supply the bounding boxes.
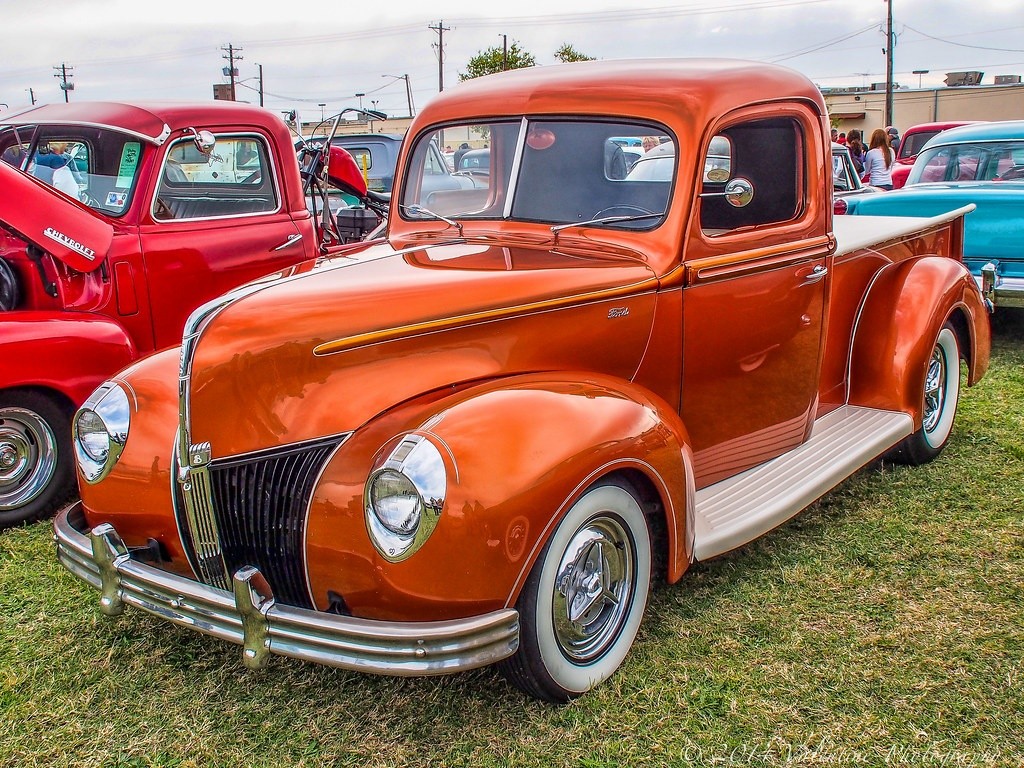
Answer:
[294,133,494,212]
[835,119,1024,308]
[890,120,1014,193]
[621,136,873,220]
[54,60,997,700]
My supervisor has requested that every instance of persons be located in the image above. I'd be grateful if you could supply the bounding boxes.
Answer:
[642,136,659,153]
[33,142,84,189]
[832,126,901,191]
[439,142,489,153]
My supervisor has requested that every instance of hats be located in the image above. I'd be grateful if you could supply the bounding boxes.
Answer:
[887,129,898,134]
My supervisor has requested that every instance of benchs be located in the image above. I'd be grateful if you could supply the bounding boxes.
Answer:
[155,195,271,218]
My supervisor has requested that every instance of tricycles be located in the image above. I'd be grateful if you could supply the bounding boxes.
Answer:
[281,108,486,251]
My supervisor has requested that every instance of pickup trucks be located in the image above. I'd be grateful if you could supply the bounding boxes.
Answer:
[0,103,380,526]
